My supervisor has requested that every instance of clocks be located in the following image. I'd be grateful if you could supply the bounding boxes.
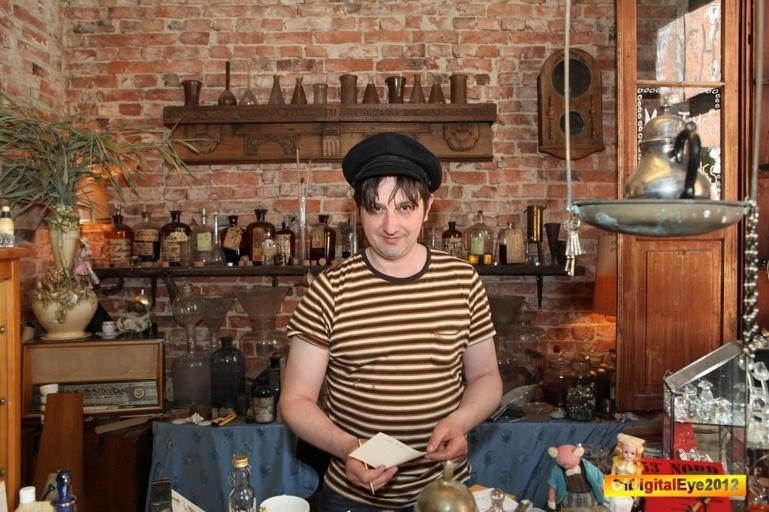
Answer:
[539,48,605,161]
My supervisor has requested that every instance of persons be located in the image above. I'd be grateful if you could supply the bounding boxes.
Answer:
[280,132,505,512]
[605,436,644,512]
[546,443,608,512]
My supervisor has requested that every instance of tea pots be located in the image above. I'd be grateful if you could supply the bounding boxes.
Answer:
[623,95,710,199]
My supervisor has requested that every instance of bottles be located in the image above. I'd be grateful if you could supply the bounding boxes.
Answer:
[0,206,14,248]
[161,210,192,269]
[132,213,160,268]
[544,355,577,408]
[342,220,360,258]
[211,336,246,418]
[594,365,609,417]
[193,207,216,266]
[55,472,76,512]
[498,220,521,266]
[243,209,277,268]
[442,221,464,266]
[252,376,275,423]
[14,487,39,512]
[310,213,336,267]
[203,211,226,268]
[565,374,597,422]
[172,338,212,414]
[229,455,256,512]
[104,214,133,269]
[251,354,284,403]
[469,210,494,269]
[604,349,617,402]
[221,215,248,266]
[274,221,296,267]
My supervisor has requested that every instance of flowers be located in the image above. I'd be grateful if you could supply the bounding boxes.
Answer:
[0,91,218,234]
[36,265,95,324]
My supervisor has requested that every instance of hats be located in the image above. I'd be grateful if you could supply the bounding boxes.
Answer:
[341,130,442,196]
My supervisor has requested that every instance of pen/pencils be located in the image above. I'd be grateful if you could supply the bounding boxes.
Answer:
[357,437,374,495]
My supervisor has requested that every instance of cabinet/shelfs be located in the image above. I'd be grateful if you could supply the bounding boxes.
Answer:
[142,403,665,512]
[163,104,497,166]
[92,265,586,310]
[20,337,164,418]
[0,247,21,511]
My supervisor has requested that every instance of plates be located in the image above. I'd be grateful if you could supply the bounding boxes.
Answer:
[94,331,122,340]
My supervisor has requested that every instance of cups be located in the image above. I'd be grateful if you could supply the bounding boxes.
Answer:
[311,82,328,103]
[102,320,116,334]
[448,71,470,103]
[259,494,310,512]
[180,78,203,108]
[338,73,359,104]
[384,76,408,104]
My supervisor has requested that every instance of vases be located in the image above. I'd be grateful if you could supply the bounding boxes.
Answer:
[32,289,97,342]
[49,229,80,272]
